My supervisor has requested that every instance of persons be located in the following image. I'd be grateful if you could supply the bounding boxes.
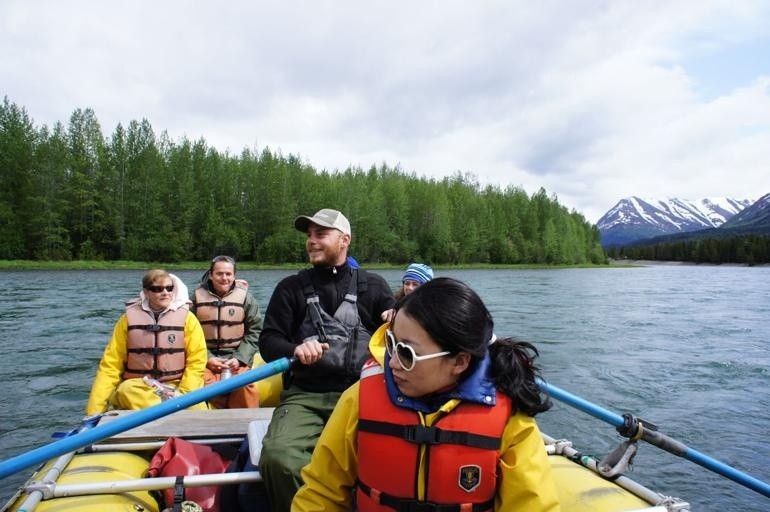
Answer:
[85,268,209,420]
[391,260,434,301]
[185,254,262,410]
[288,276,565,511]
[257,208,402,511]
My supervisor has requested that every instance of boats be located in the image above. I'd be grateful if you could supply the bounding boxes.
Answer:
[7,343,699,512]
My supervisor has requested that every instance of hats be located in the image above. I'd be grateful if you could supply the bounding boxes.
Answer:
[294,207,351,235]
[402,263,434,285]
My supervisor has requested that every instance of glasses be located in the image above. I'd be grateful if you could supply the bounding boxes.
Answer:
[384,329,452,371]
[148,285,174,293]
